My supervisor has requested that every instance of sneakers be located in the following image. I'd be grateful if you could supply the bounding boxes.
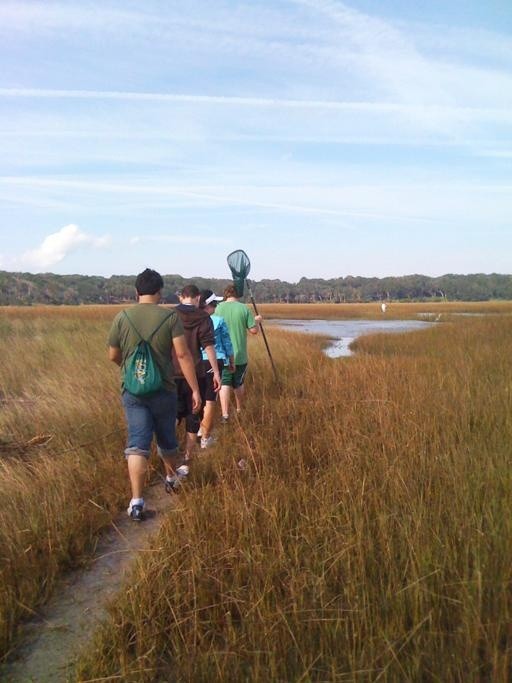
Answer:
[165,415,231,494]
[127,497,145,520]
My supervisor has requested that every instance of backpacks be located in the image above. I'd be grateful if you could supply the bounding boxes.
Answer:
[122,308,174,396]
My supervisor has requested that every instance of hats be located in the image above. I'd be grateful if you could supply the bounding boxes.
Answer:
[201,293,224,304]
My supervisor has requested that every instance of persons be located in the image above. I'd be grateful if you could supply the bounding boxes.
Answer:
[172,283,263,461]
[105,267,203,521]
